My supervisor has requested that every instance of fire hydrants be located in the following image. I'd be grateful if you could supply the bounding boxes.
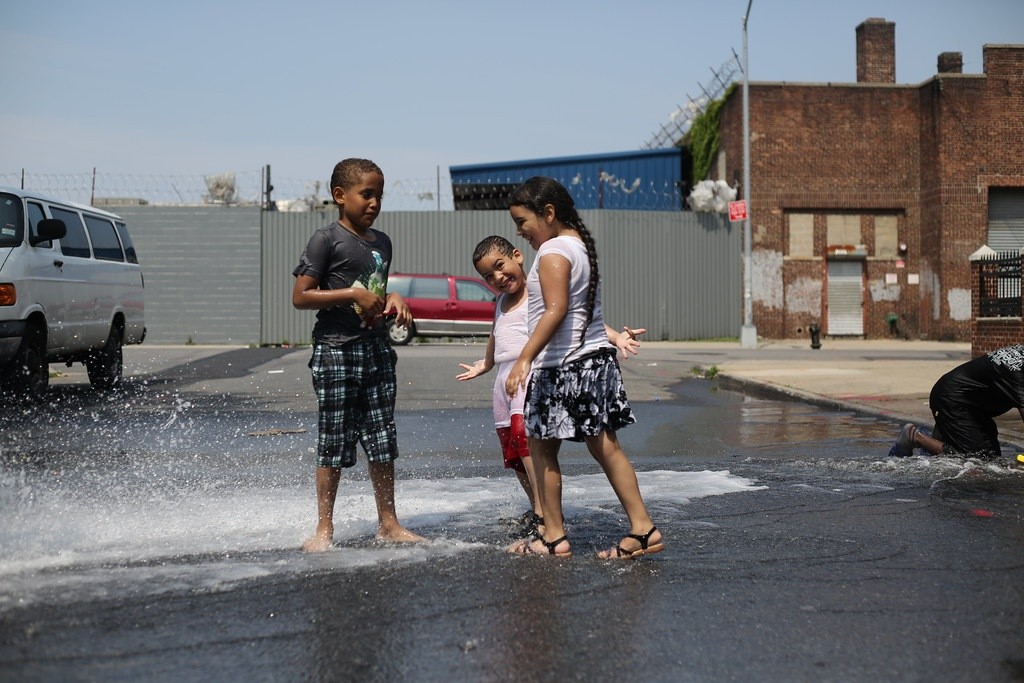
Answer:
[808,321,822,350]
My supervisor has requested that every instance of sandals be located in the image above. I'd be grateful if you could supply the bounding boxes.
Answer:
[506,509,564,541]
[508,535,571,558]
[598,526,665,560]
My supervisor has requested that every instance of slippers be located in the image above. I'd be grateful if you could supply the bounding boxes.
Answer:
[888,424,913,458]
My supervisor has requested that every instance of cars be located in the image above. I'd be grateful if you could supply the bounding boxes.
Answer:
[387,271,502,347]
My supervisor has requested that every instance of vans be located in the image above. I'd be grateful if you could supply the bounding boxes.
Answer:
[0,184,146,409]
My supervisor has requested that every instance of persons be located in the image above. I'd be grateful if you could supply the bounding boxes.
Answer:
[454,232,644,552]
[888,341,1024,464]
[504,176,667,561]
[292,155,434,553]
